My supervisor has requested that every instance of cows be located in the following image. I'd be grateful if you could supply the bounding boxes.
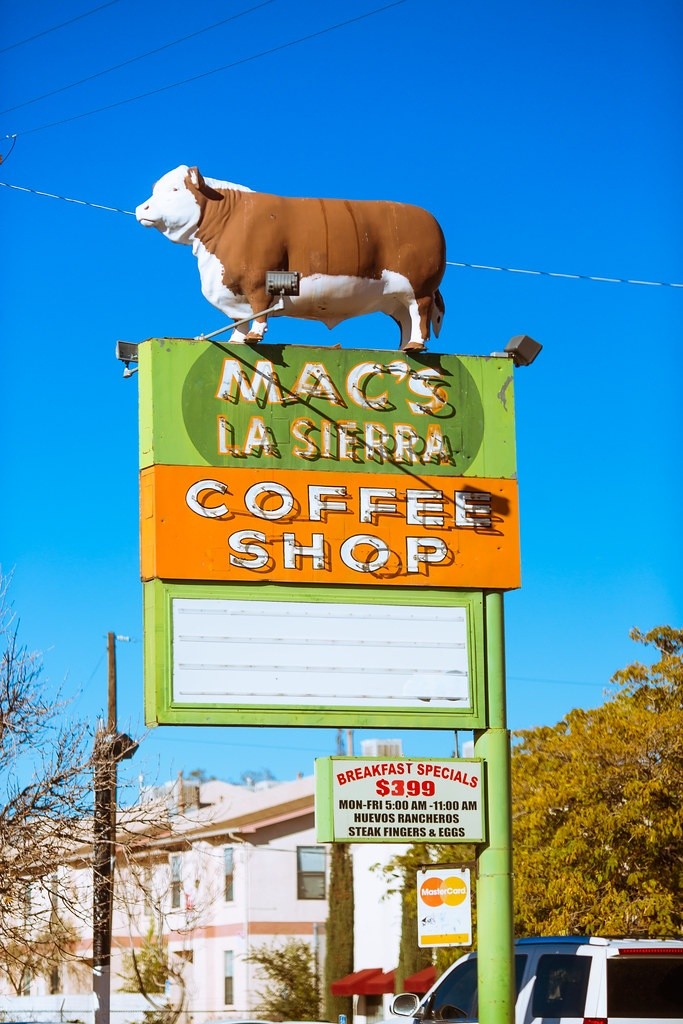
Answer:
[136,165,447,353]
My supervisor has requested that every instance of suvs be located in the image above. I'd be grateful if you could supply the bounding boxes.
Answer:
[388,934,683,1024]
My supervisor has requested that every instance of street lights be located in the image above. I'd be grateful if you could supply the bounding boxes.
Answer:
[89,729,141,1024]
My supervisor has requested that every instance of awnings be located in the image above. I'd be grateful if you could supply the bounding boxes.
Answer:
[330,965,438,997]
